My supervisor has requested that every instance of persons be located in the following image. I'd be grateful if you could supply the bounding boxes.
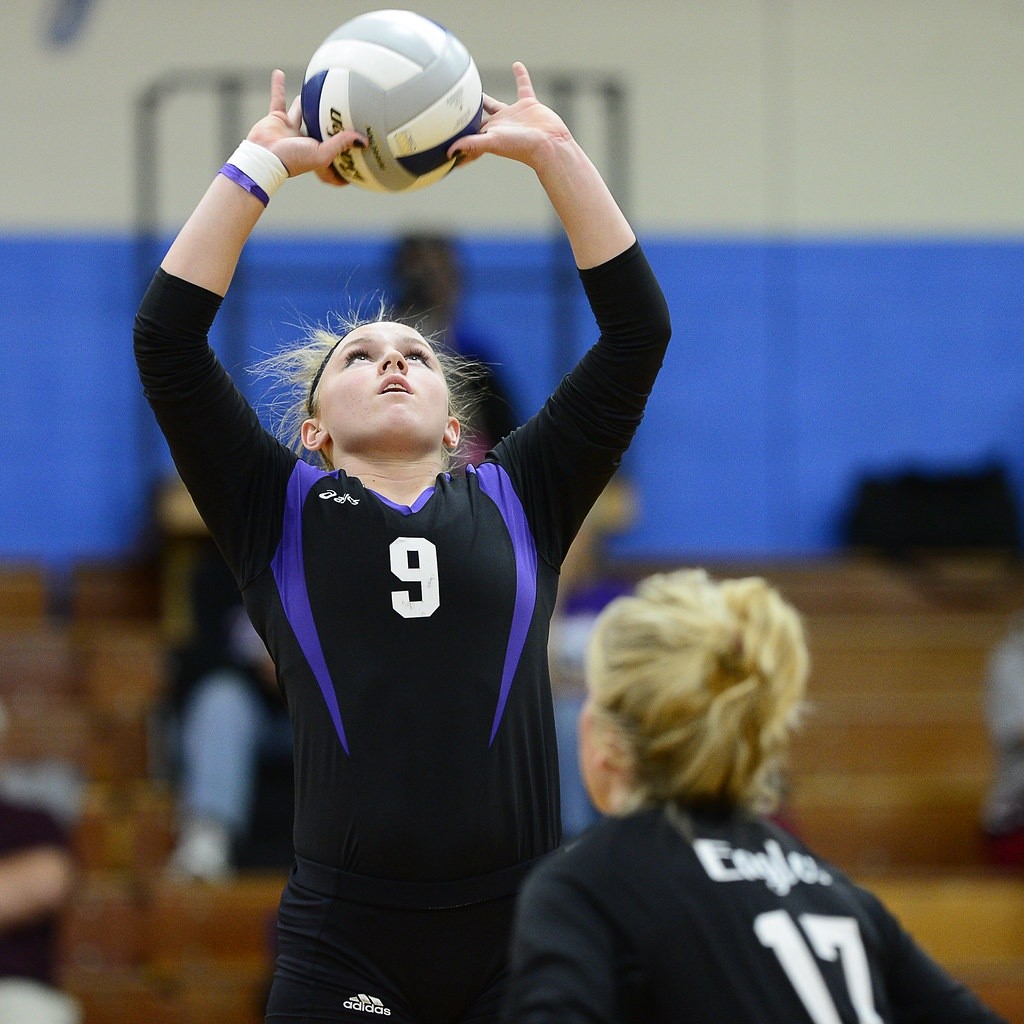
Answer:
[387,233,520,466]
[0,793,85,1021]
[508,567,1012,1024]
[131,59,674,1024]
[179,596,298,877]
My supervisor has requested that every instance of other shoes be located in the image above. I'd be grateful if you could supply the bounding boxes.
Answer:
[170,844,234,884]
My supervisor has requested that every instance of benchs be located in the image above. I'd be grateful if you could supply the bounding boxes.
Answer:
[0,550,1024,1024]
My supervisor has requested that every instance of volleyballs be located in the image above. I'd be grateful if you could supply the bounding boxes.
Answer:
[301,8,483,192]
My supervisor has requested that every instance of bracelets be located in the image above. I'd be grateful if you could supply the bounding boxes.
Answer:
[222,137,292,208]
